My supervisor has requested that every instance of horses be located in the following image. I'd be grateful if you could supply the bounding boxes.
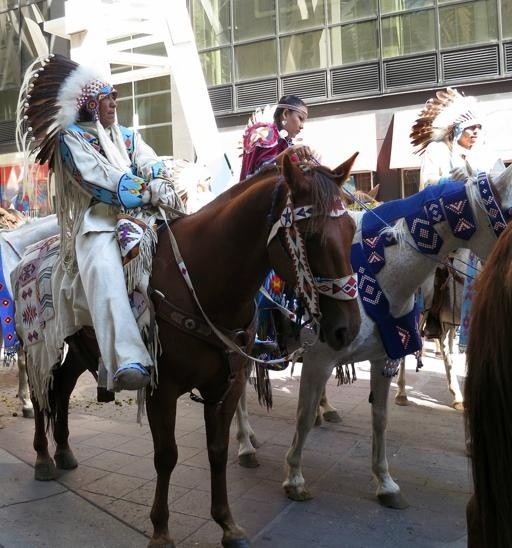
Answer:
[0,213,64,418]
[461,216,512,548]
[313,160,508,424]
[12,150,361,548]
[339,182,382,205]
[237,159,512,511]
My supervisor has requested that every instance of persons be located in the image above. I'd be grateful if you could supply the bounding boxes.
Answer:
[410,88,482,337]
[239,95,313,353]
[15,52,174,388]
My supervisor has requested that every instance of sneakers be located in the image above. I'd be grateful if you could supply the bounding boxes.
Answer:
[113,362,152,391]
